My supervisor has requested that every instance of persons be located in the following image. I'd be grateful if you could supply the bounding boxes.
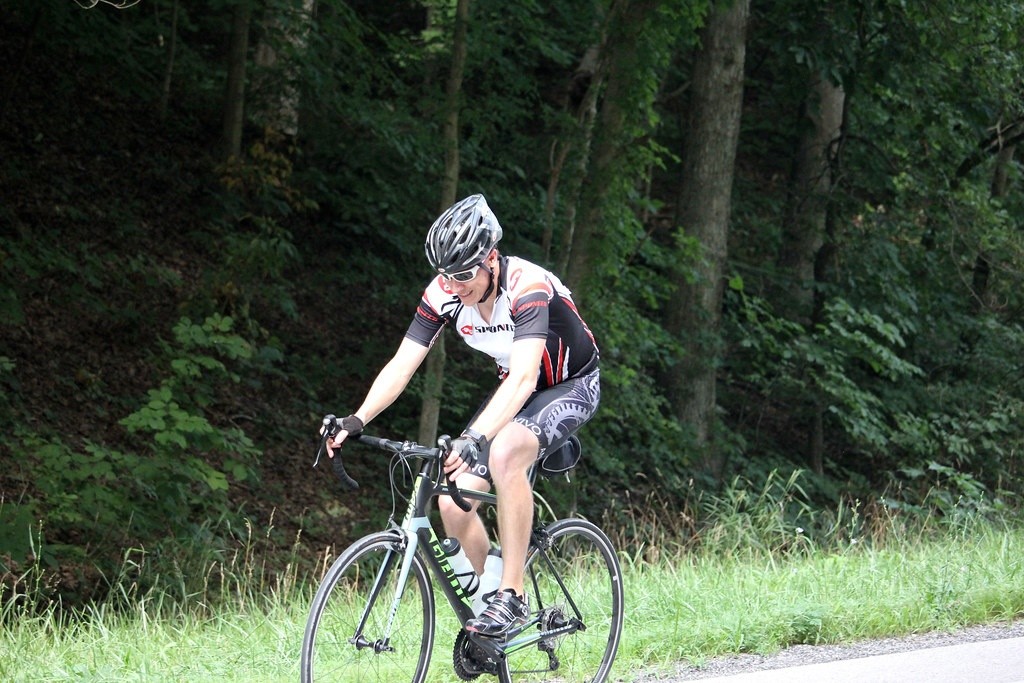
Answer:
[320,193,601,636]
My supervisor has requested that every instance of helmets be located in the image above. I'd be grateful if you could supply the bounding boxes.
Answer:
[426,194,503,273]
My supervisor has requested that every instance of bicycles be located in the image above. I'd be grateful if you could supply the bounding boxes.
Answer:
[301,413,625,683]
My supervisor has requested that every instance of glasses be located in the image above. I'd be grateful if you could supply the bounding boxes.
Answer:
[442,259,486,283]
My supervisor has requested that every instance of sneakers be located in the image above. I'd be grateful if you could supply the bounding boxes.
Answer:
[465,591,530,636]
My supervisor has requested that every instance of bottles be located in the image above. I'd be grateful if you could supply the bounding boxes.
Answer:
[441,537,482,600]
[470,546,503,618]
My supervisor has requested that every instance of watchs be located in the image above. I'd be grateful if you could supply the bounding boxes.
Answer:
[462,428,488,453]
